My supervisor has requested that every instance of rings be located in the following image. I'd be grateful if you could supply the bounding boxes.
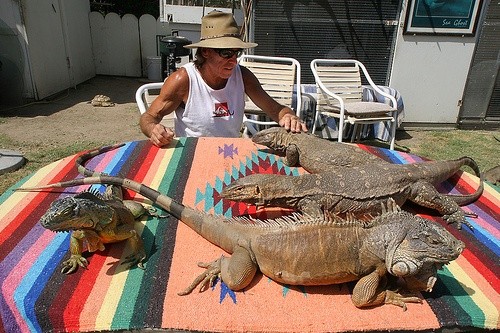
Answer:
[293,117,299,121]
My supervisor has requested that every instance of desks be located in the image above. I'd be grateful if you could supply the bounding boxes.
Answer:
[0,137,500,333]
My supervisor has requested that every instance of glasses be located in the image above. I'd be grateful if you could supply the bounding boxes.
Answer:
[213,48,244,58]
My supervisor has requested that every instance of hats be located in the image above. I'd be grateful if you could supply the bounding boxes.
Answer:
[183,10,258,49]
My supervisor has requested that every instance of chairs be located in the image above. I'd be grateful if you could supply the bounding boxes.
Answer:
[135,82,257,137]
[310,59,398,150]
[238,54,301,136]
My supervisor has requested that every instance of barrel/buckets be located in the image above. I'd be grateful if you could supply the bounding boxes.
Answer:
[143,56,162,81]
[159,35,192,83]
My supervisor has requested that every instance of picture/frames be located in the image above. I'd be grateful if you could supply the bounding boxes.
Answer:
[402,0,484,38]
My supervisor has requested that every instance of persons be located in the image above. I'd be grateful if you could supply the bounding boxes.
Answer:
[139,10,308,148]
